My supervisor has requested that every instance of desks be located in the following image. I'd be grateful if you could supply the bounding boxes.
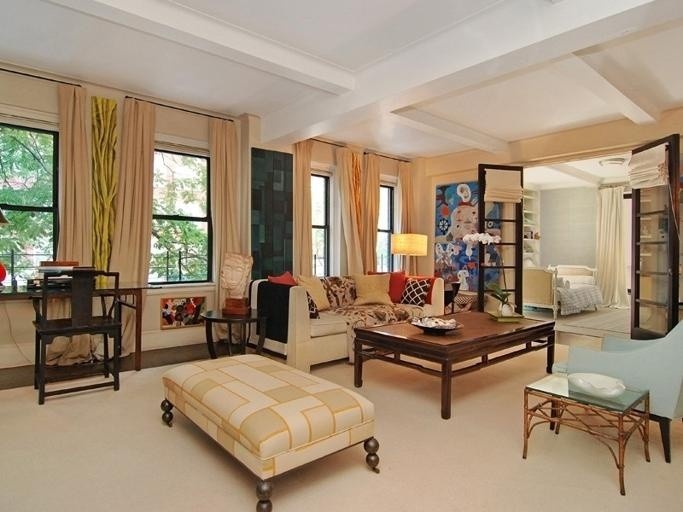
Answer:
[0,280,163,372]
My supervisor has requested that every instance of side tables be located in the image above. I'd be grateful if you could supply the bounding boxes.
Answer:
[201,308,266,360]
[522,372,650,495]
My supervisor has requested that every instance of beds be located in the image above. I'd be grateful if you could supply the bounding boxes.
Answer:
[522,264,598,321]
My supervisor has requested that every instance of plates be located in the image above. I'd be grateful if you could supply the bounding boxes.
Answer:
[488,311,525,323]
[411,317,464,336]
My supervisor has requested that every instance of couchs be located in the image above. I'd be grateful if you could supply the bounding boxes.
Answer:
[161,351,380,512]
[549,319,683,462]
[250,274,443,373]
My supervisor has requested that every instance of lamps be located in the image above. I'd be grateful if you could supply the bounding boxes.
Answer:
[599,158,625,178]
[390,232,428,275]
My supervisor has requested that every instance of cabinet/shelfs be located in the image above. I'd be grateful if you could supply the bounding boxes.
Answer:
[523,186,541,266]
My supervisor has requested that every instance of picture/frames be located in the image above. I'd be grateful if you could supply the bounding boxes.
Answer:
[160,296,207,329]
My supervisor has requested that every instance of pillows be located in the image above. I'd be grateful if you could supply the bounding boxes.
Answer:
[296,275,330,311]
[350,273,395,307]
[267,271,297,286]
[400,277,432,307]
[368,270,405,303]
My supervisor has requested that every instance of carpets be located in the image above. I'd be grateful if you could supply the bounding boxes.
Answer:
[522,305,631,338]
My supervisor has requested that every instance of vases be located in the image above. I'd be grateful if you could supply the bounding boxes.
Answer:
[502,304,514,317]
